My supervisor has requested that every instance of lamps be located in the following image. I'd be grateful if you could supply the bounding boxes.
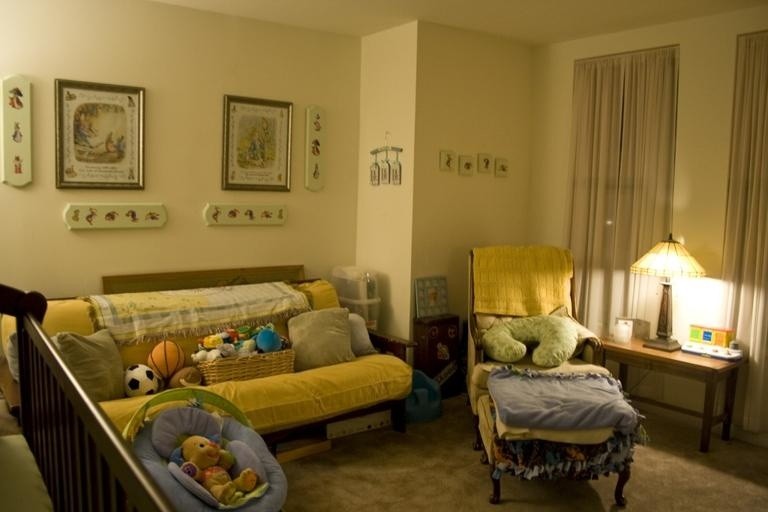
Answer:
[629,231,705,352]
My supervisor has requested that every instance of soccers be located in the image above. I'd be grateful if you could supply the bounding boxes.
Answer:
[124,363,158,397]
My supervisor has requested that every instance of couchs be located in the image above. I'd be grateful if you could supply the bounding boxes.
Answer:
[3,276,417,458]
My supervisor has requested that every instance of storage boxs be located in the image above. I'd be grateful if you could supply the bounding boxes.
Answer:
[328,266,382,334]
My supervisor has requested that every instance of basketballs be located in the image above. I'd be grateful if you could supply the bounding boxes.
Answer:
[147,341,185,380]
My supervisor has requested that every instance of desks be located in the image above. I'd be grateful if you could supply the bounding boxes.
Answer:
[597,337,748,453]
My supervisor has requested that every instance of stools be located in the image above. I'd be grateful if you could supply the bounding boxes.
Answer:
[473,385,638,508]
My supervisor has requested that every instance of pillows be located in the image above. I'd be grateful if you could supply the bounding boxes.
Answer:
[6,329,59,382]
[288,302,354,372]
[479,313,581,369]
[56,322,127,403]
[345,312,378,357]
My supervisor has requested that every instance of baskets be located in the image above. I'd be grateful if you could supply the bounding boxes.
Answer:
[197,348,296,386]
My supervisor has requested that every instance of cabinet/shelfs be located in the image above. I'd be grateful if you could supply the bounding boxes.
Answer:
[413,312,460,400]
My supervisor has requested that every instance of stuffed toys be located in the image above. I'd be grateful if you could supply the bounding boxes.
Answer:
[167,431,258,505]
[169,365,201,388]
[190,322,289,364]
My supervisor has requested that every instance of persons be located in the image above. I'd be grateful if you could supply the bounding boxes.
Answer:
[78,111,116,154]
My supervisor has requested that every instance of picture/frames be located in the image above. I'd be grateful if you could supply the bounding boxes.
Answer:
[102,264,307,296]
[414,275,450,316]
[369,161,402,186]
[221,92,292,192]
[439,147,511,179]
[614,317,636,344]
[53,76,146,190]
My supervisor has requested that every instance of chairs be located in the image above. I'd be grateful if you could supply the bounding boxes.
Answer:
[467,246,604,448]
[119,385,288,512]
